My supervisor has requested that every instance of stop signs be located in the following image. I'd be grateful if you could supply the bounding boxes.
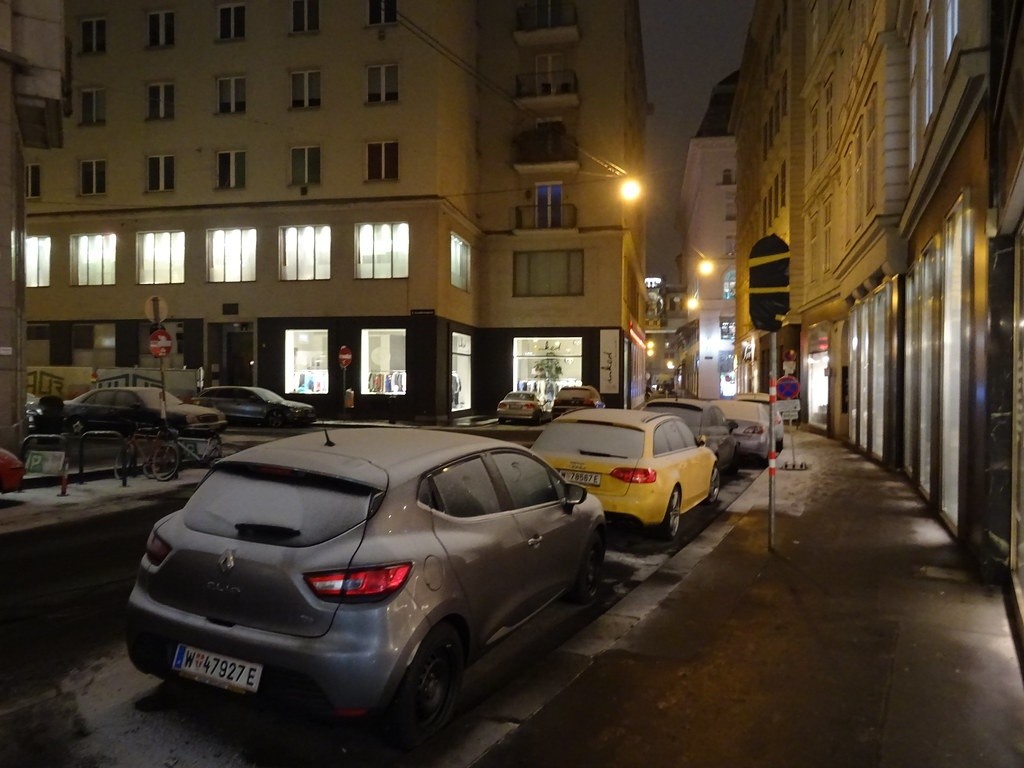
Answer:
[338,345,352,369]
[148,330,171,358]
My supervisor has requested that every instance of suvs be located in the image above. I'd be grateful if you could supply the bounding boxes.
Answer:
[552,386,607,422]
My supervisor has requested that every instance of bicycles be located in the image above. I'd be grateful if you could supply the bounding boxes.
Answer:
[146,425,224,481]
[114,418,184,480]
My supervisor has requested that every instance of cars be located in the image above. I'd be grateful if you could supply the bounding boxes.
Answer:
[640,395,741,476]
[497,391,544,426]
[530,408,720,540]
[189,385,318,427]
[124,415,608,752]
[732,392,785,454]
[0,447,26,497]
[58,386,228,440]
[709,400,772,467]
[24,391,64,442]
